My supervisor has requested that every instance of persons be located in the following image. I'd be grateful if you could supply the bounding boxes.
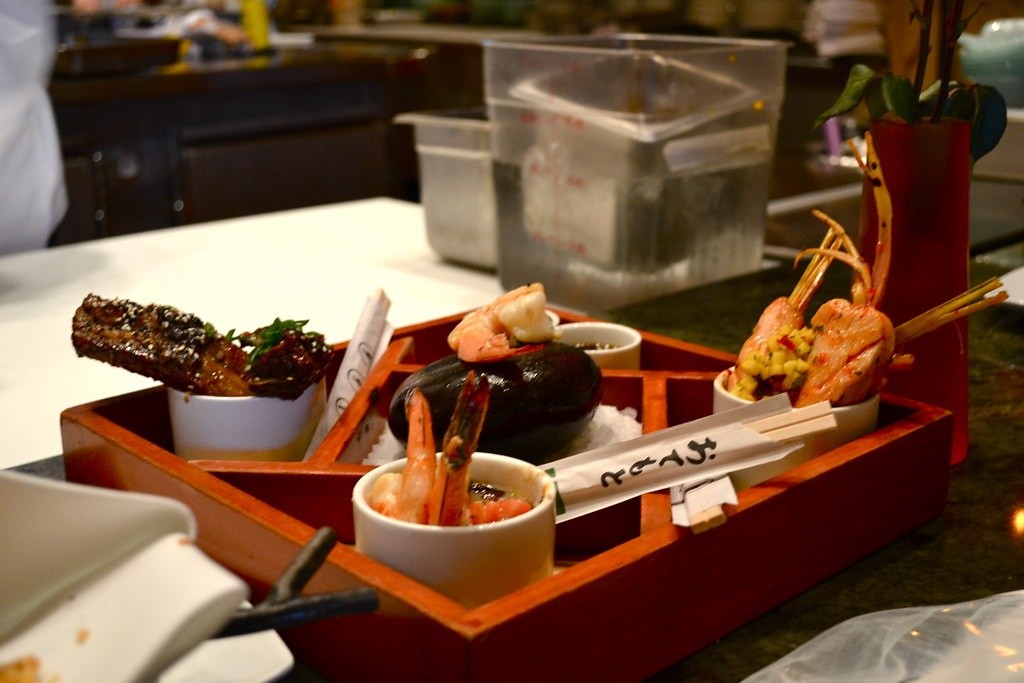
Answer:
[0,0,174,256]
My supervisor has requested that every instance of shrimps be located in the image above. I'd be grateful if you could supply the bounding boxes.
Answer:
[372,134,913,527]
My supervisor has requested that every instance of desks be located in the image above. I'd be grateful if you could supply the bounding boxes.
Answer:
[0,195,587,469]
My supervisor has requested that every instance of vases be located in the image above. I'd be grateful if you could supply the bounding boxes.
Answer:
[850,117,970,469]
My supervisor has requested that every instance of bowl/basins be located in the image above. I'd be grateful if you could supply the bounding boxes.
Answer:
[392,35,790,314]
[355,451,558,608]
[713,371,883,493]
[527,309,644,372]
[167,377,327,466]
[387,341,602,468]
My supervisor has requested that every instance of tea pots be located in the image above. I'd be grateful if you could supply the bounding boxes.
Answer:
[953,19,1023,110]
